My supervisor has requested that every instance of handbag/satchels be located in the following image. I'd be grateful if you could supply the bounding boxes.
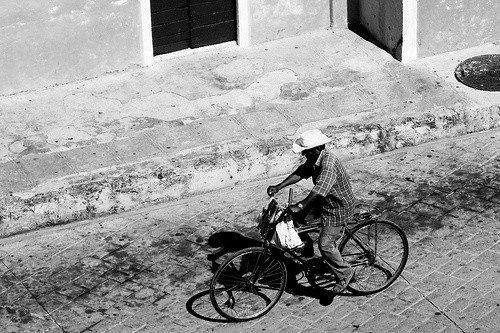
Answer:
[274,211,305,249]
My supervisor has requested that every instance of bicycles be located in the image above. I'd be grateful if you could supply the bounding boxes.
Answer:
[209,192,409,321]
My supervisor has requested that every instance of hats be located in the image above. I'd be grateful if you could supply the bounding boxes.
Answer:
[292,129,332,153]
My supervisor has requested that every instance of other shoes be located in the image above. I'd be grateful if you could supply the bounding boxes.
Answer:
[333,268,355,293]
[284,249,315,259]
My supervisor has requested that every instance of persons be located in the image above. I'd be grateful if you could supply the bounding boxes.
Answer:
[267,128,355,292]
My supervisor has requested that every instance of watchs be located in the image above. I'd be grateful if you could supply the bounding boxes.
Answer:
[297,202,304,210]
